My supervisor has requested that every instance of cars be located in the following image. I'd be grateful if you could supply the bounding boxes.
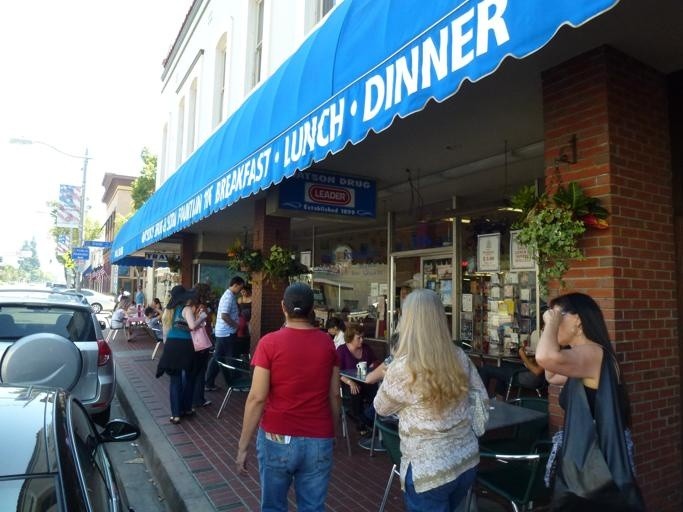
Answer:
[0,278,144,512]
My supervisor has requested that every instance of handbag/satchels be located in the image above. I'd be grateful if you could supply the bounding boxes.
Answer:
[548,345,649,512]
[470,389,488,437]
[189,314,212,352]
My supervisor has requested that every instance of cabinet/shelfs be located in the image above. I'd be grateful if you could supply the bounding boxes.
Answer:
[420,253,452,316]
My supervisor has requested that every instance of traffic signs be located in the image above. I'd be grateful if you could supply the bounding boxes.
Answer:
[84,240,110,248]
[71,247,89,260]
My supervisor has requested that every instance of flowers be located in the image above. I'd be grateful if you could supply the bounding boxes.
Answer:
[225,236,265,275]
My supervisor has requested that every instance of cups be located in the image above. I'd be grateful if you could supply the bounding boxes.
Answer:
[355,360,365,376]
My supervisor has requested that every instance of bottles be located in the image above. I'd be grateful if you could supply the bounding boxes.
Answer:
[198,308,205,328]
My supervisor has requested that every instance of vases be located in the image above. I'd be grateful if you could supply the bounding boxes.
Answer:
[240,261,253,272]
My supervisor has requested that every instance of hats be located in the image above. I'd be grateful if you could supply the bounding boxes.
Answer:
[165,285,198,309]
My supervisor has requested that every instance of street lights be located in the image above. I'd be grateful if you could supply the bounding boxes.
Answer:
[10,136,89,294]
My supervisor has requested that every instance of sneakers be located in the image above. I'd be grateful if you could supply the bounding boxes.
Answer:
[169,383,252,424]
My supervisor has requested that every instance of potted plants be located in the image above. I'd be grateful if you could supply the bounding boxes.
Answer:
[167,255,182,273]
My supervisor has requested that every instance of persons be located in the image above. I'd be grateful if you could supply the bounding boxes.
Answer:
[529,312,545,352]
[480,346,547,387]
[373,288,490,512]
[237,283,341,512]
[162,276,252,425]
[535,291,645,512]
[111,286,162,342]
[326,317,400,438]
